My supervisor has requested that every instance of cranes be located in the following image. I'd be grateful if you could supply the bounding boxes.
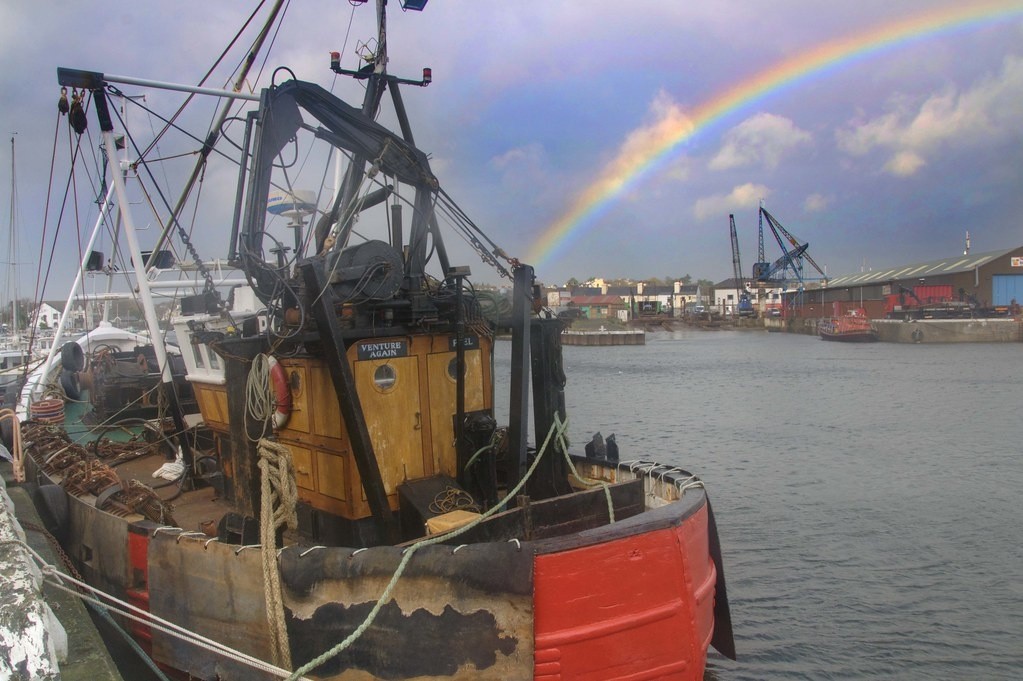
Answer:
[729,213,756,317]
[750,199,828,288]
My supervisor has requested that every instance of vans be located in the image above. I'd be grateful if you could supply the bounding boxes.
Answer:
[694,305,705,314]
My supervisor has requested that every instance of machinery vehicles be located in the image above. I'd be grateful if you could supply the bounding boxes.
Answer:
[886,274,982,319]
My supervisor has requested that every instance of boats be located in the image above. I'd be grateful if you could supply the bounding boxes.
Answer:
[817,307,879,343]
[0,1,740,681]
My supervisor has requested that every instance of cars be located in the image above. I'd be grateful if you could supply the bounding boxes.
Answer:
[767,307,780,317]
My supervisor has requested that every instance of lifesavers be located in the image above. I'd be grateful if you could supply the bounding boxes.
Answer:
[61,370,82,400]
[912,330,923,341]
[61,342,84,370]
[267,354,291,431]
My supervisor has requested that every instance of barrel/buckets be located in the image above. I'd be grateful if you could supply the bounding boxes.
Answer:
[30,399,65,430]
